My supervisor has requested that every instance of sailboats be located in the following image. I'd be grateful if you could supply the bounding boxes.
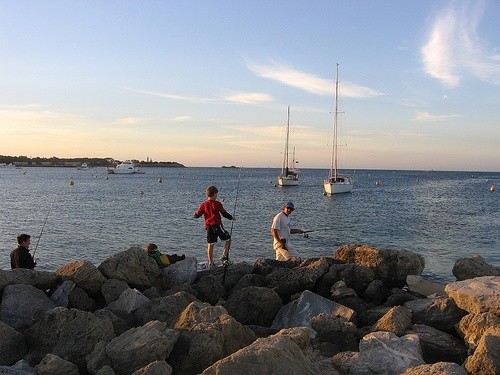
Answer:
[324,62,353,194]
[278,105,300,186]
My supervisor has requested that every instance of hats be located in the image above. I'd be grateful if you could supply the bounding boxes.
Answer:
[16,234,31,243]
[284,201,295,208]
[148,244,158,251]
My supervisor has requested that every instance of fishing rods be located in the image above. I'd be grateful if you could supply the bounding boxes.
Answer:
[32,200,55,259]
[299,223,373,240]
[220,163,242,286]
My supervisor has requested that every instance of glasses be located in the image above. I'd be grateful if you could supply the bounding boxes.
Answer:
[287,207,294,211]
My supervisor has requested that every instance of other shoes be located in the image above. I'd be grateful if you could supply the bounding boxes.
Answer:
[207,262,218,269]
[219,257,234,266]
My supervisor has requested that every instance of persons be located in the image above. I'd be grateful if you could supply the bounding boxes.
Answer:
[193,186,236,266]
[10,234,37,270]
[146,243,186,267]
[270,202,303,261]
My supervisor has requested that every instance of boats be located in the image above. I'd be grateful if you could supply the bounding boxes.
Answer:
[0,163,26,175]
[77,167,89,170]
[106,161,146,174]
